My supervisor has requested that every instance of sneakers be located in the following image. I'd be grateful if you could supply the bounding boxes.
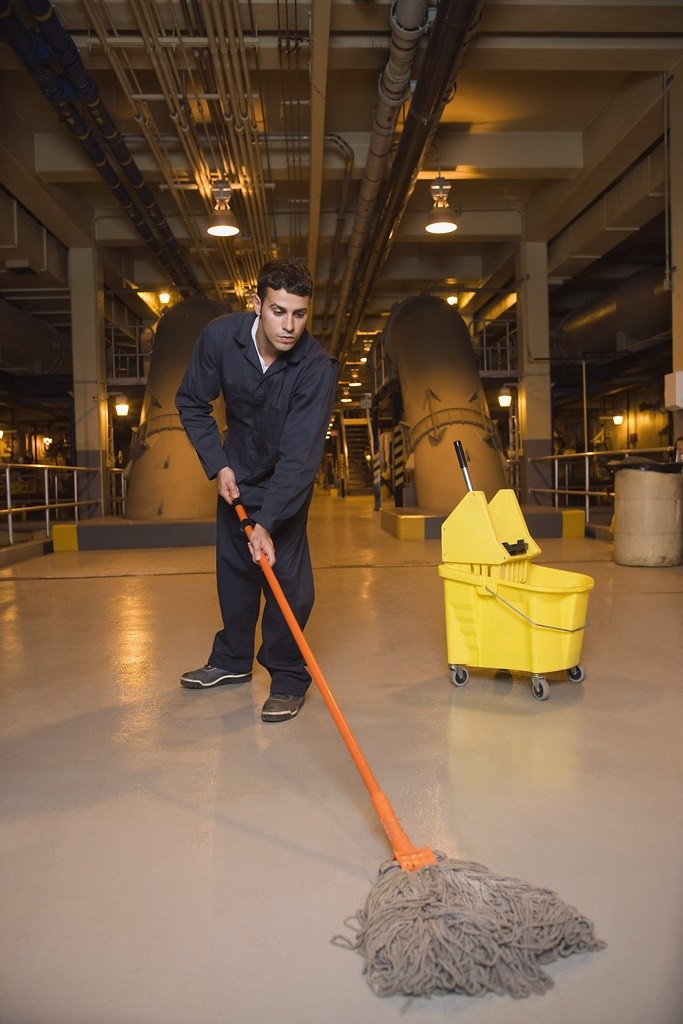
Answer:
[179,664,252,689]
[260,692,305,722]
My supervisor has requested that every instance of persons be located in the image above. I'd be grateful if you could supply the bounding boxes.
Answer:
[176,259,338,722]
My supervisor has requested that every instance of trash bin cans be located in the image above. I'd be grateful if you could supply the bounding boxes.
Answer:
[613,456,683,567]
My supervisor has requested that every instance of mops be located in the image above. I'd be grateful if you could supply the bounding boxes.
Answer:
[227,492,608,1000]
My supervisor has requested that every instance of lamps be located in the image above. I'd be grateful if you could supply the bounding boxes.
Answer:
[425,164,458,234]
[611,408,624,426]
[206,179,240,237]
[108,391,130,416]
[497,382,518,407]
